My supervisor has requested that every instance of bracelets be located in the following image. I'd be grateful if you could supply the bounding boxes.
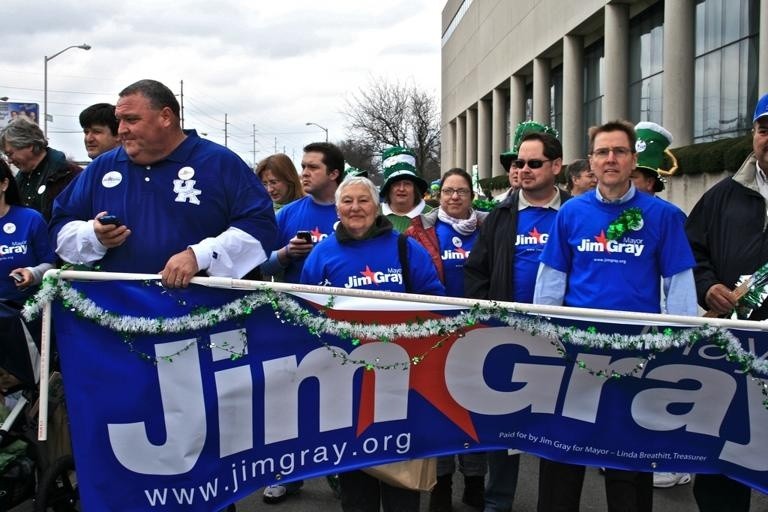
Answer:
[284,246,289,260]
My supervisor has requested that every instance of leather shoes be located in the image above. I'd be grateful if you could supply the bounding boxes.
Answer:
[326,475,343,499]
[263,478,304,505]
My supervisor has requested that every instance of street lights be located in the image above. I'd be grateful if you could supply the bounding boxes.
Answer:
[306,122,329,143]
[42,44,92,139]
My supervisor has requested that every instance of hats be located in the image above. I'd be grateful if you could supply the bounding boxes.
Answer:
[499,121,559,173]
[344,159,368,178]
[378,146,428,198]
[752,92,768,123]
[634,121,672,172]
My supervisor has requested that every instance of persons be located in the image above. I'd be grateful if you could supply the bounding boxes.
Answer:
[78,103,121,159]
[630,121,691,487]
[495,122,559,201]
[461,132,575,511]
[298,174,446,512]
[49,77,277,512]
[260,142,344,504]
[406,169,493,512]
[532,120,697,512]
[0,154,56,396]
[683,93,767,512]
[252,153,303,213]
[564,159,597,197]
[0,117,82,224]
[376,146,434,233]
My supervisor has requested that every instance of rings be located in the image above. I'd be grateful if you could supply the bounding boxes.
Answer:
[176,278,182,283]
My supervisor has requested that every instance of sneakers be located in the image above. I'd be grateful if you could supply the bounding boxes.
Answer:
[652,471,691,488]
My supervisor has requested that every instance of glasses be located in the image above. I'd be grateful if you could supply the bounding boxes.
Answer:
[577,174,594,178]
[441,189,471,196]
[515,159,551,169]
[592,147,631,157]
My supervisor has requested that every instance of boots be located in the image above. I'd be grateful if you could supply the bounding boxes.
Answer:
[429,473,455,512]
[462,476,485,507]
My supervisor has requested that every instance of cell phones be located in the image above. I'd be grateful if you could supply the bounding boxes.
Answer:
[99,215,119,226]
[13,273,23,281]
[297,231,312,244]
